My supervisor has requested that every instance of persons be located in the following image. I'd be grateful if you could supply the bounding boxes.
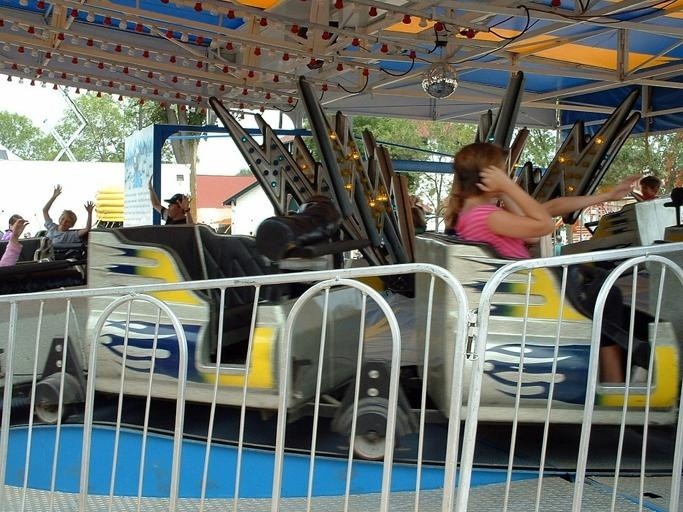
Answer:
[628,176,661,201]
[379,190,432,234]
[0,213,24,241]
[41,183,95,261]
[443,170,643,236]
[147,173,194,226]
[451,141,643,383]
[0,219,29,267]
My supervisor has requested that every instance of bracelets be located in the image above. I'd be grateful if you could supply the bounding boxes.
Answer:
[184,208,189,213]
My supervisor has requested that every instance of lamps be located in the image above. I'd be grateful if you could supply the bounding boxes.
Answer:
[422,38,459,100]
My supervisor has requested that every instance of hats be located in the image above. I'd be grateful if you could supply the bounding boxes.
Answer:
[164,193,190,211]
